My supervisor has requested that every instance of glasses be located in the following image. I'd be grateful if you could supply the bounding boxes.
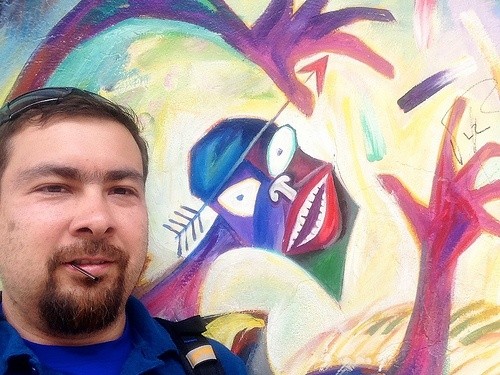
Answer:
[1,87,119,124]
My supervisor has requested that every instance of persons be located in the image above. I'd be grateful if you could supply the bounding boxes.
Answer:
[0,84,251,375]
